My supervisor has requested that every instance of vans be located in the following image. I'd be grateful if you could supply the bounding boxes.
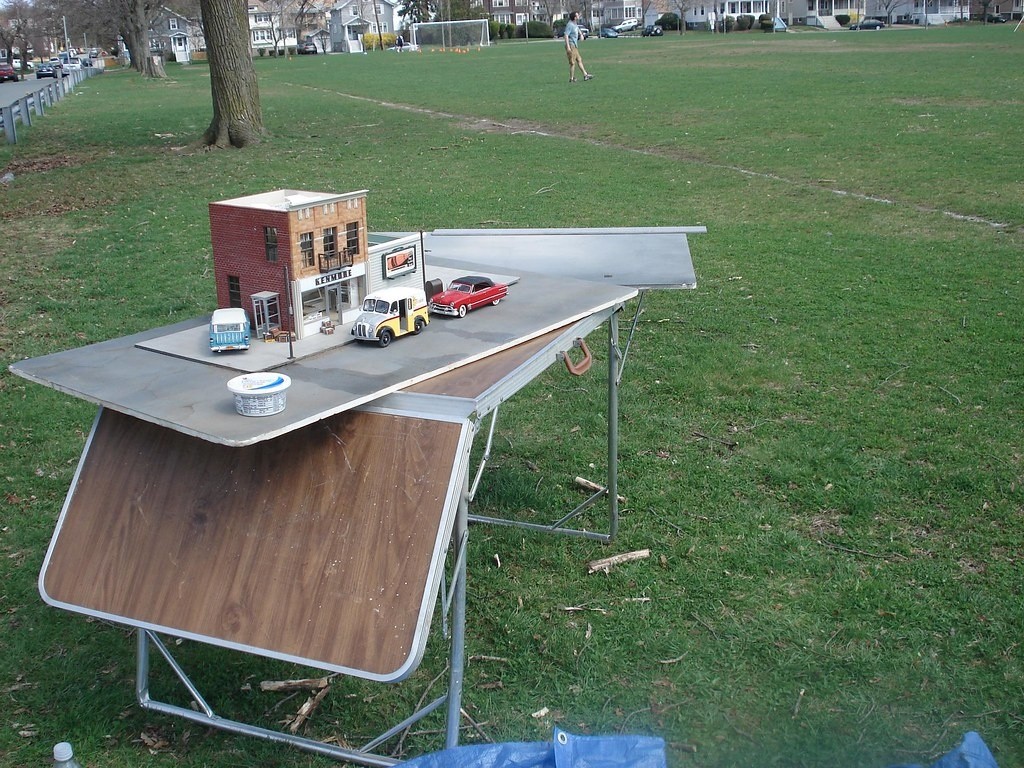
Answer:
[60,57,85,78]
[351,287,430,348]
[209,308,251,352]
[57,53,68,66]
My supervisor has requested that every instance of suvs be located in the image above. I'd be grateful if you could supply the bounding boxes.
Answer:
[641,25,663,37]
[0,65,18,83]
[611,19,638,33]
[49,57,62,68]
[300,43,317,54]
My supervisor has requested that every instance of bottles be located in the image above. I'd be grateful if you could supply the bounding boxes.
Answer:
[51,742,82,768]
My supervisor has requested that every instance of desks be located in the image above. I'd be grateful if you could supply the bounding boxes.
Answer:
[37,256,643,768]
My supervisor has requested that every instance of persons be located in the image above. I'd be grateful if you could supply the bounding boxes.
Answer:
[564,10,596,83]
[396,33,404,52]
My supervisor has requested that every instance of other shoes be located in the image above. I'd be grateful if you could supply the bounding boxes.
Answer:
[584,74,594,81]
[570,77,577,82]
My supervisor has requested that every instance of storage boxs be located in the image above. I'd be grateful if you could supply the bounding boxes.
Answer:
[320,319,336,334]
[263,328,296,342]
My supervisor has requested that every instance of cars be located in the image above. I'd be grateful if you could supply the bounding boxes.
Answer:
[36,63,58,79]
[428,276,509,318]
[557,25,589,40]
[849,18,885,30]
[597,28,618,38]
[979,12,1008,24]
[386,42,420,51]
[69,48,98,58]
[77,54,92,66]
[0,59,34,71]
